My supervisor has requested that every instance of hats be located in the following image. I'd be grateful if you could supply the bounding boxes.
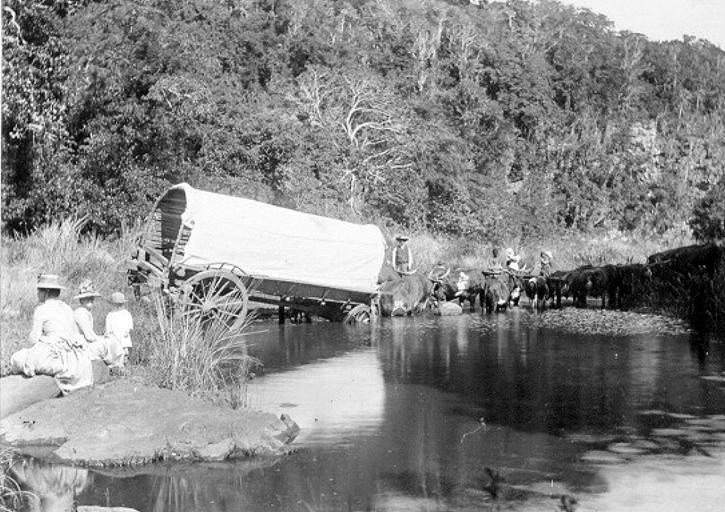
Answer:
[36,274,62,289]
[396,236,409,241]
[106,292,129,305]
[73,284,102,300]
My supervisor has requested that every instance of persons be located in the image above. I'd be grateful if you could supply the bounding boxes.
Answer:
[391,233,414,278]
[104,292,134,362]
[540,250,554,277]
[504,247,521,271]
[72,281,123,372]
[7,274,79,377]
[490,248,501,276]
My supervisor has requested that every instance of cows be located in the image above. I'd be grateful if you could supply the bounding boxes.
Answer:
[647,242,722,279]
[377,262,657,319]
